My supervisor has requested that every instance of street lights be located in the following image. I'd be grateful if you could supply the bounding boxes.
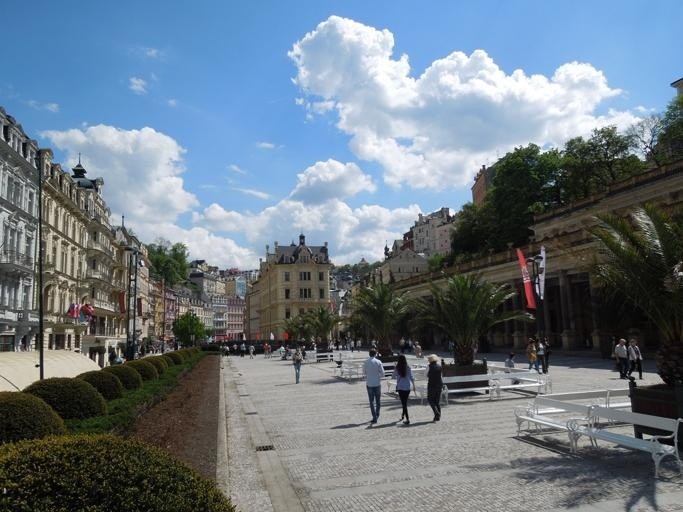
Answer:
[526,255,543,336]
[123,245,138,359]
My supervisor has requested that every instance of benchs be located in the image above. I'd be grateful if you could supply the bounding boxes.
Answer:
[308,351,548,397]
[528,388,608,434]
[608,387,633,427]
[571,404,683,480]
[515,395,595,461]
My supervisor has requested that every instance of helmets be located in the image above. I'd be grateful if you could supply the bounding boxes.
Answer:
[427,353,439,363]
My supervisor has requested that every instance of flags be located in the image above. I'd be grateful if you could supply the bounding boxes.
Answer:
[65,303,94,323]
[517,250,537,309]
[535,246,546,300]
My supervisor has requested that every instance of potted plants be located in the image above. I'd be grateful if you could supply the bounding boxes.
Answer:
[553,200,682,456]
[301,267,536,396]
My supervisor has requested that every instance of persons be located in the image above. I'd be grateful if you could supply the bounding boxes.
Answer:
[440,359,446,365]
[526,337,551,375]
[221,342,246,358]
[329,334,362,353]
[108,338,180,365]
[399,336,422,358]
[263,342,272,359]
[392,354,416,424]
[424,354,443,421]
[278,340,307,384]
[248,344,255,359]
[505,353,519,384]
[362,348,385,423]
[614,338,643,381]
